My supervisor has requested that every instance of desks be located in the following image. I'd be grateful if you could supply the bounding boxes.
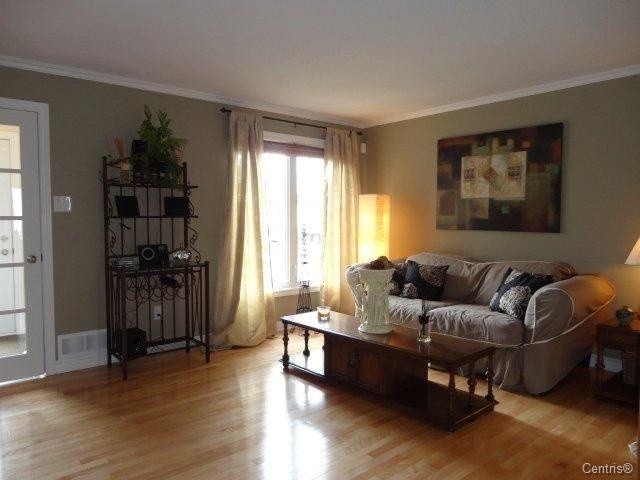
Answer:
[597,316,640,411]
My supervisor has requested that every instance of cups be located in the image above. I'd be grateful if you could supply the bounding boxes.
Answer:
[317,305,331,321]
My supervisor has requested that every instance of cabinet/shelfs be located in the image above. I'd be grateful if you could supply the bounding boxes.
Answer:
[102,157,210,380]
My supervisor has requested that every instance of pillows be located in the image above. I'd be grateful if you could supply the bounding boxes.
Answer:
[369,255,552,318]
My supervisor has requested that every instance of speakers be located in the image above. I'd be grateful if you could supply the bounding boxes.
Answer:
[115,327,147,358]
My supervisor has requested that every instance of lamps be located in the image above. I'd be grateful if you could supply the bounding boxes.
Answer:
[625,237,640,265]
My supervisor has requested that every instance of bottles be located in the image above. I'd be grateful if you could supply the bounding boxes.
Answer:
[120,157,131,184]
[171,243,191,266]
[416,315,432,342]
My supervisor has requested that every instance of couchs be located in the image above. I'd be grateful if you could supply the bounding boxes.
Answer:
[345,252,617,397]
